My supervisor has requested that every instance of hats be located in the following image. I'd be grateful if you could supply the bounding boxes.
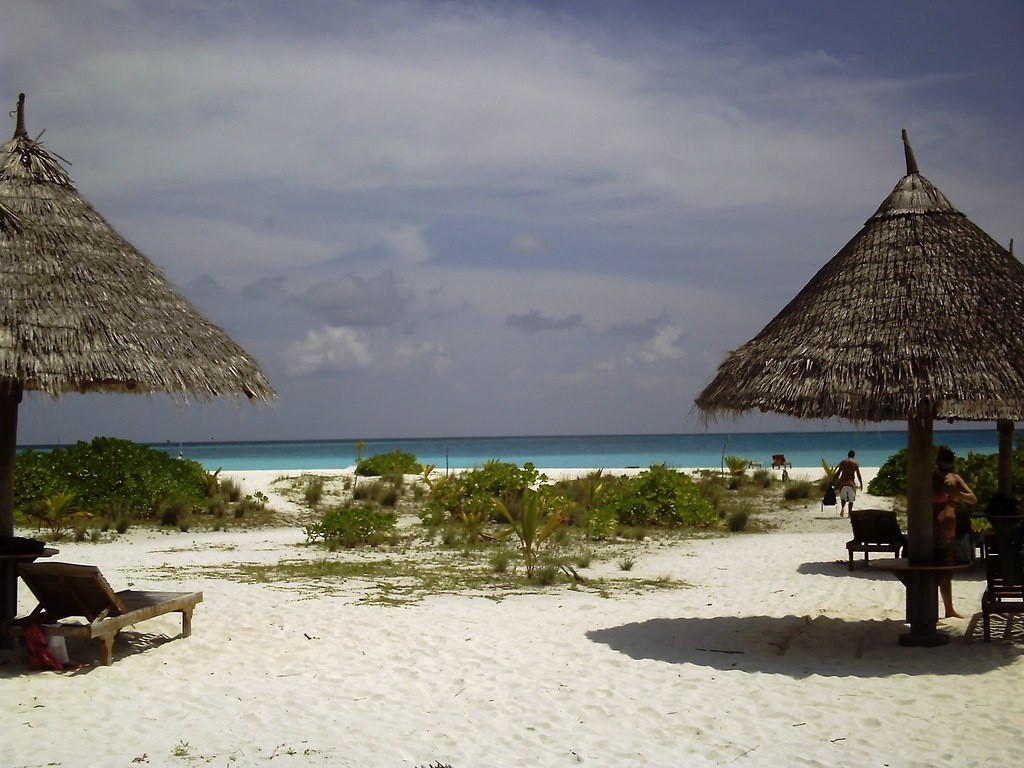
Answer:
[937,449,955,465]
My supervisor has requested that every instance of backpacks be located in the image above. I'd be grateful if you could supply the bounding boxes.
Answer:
[822,486,836,506]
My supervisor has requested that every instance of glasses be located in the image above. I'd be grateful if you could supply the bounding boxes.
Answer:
[939,464,954,473]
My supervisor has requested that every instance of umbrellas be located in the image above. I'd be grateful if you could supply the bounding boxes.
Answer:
[695,125,1024,650]
[0,94,275,546]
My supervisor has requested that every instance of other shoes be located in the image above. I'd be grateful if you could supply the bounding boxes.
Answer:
[840,509,844,517]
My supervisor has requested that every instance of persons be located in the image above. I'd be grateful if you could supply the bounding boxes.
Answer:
[929,444,977,618]
[829,450,863,518]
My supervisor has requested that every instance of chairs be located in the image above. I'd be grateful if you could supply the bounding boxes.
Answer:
[846,509,909,572]
[6,562,204,665]
[957,511,975,561]
[771,454,792,469]
[982,533,1024,644]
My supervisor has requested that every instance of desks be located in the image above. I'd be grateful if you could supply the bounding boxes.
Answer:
[0,548,60,651]
[868,558,977,648]
